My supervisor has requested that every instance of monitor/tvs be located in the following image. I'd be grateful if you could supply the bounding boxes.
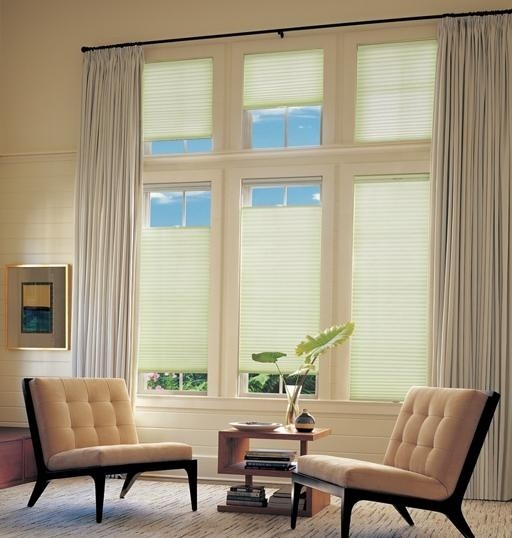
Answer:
[226,485,307,511]
[244,449,298,470]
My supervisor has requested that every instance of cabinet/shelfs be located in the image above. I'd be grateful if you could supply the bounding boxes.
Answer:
[295,408,315,433]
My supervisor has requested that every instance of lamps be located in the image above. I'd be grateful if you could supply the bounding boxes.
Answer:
[291,386,500,538]
[22,378,197,524]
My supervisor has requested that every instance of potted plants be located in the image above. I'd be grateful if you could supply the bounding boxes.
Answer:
[4,264,69,350]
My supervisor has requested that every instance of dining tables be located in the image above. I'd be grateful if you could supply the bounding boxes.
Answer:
[252,322,354,428]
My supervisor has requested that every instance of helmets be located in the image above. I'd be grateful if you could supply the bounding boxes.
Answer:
[230,420,283,432]
